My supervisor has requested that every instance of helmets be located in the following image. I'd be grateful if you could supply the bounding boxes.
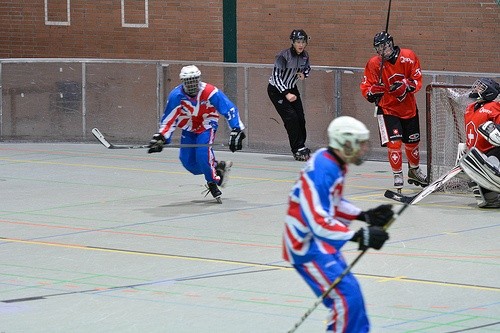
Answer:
[290,29,308,43]
[327,116,370,150]
[373,31,395,60]
[468,76,500,101]
[180,65,201,96]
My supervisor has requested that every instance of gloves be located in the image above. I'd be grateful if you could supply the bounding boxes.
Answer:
[228,127,245,153]
[388,77,416,98]
[358,226,389,251]
[148,133,166,153]
[358,204,395,227]
[367,83,386,101]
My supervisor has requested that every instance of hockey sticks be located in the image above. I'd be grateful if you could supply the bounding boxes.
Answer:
[286,188,422,333]
[383,165,464,206]
[372,0,392,87]
[91,127,230,150]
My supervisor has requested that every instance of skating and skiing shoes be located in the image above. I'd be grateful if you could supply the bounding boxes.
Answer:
[293,148,311,162]
[202,182,222,204]
[215,160,235,187]
[394,172,404,194]
[408,162,427,187]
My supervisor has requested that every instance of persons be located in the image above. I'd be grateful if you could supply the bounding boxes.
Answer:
[282,116,394,333]
[267,29,311,161]
[457,77,500,207]
[148,64,246,204]
[360,31,431,196]
